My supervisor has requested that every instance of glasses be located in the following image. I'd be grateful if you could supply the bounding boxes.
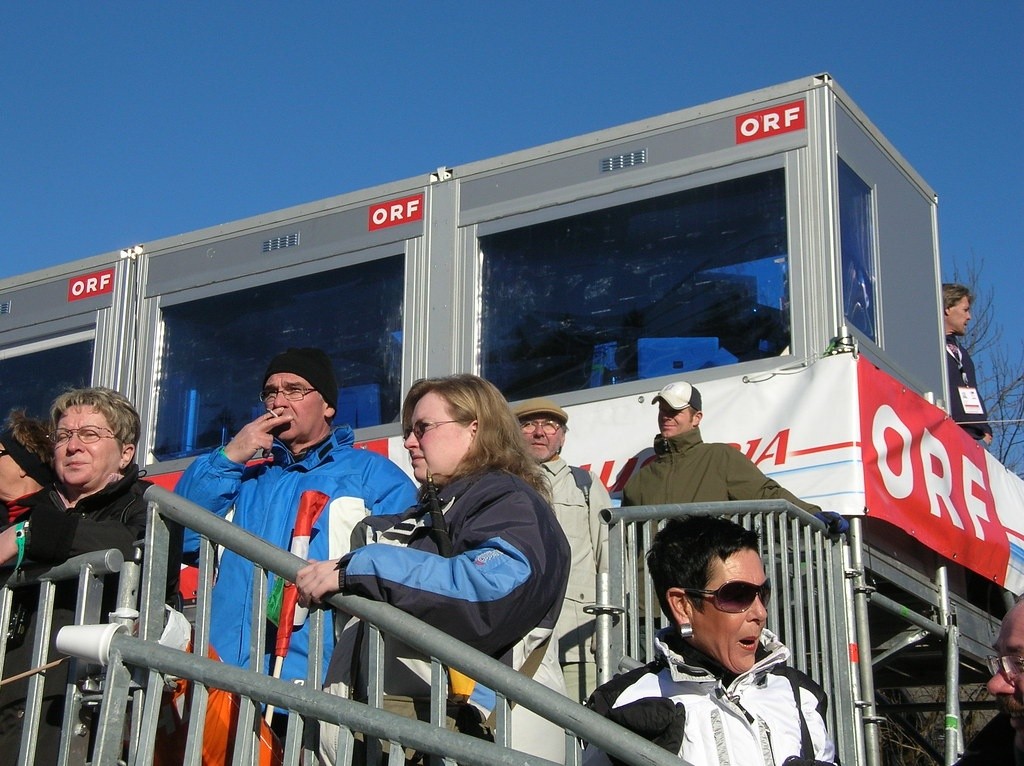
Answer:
[46,425,126,446]
[403,419,475,441]
[985,655,1024,682]
[259,386,316,402]
[663,578,771,612]
[519,420,562,435]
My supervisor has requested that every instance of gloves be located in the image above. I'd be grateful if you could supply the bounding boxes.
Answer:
[814,511,849,534]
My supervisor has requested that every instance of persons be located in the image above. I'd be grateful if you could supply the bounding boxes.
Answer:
[0,387,184,766]
[513,398,632,704]
[174,346,420,739]
[295,372,571,766]
[621,381,849,664]
[580,513,836,766]
[941,283,992,450]
[782,247,874,346]
[952,592,1024,766]
[0,405,55,528]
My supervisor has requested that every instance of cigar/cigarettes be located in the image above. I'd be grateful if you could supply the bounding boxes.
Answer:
[267,408,278,418]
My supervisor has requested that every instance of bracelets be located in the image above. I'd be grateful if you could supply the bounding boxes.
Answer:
[14,520,25,570]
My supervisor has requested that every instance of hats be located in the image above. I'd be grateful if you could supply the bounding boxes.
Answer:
[512,398,568,424]
[263,347,337,419]
[652,381,701,411]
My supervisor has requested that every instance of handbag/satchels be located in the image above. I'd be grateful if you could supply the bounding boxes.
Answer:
[354,695,497,766]
